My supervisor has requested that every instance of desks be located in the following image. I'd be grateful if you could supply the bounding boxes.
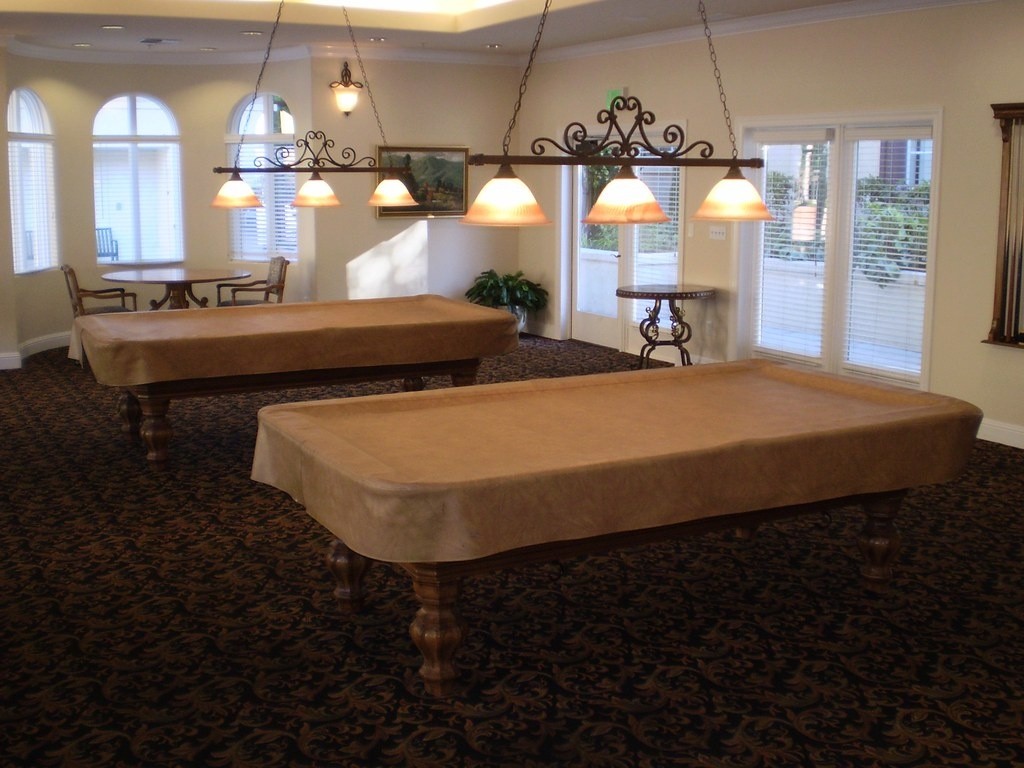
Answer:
[101,269,252,312]
[251,357,985,699]
[68,293,520,462]
[615,283,717,370]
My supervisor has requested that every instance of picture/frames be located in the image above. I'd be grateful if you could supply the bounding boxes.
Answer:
[374,144,469,219]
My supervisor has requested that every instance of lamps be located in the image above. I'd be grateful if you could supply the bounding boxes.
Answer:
[327,61,364,117]
[460,0,777,224]
[214,1,422,208]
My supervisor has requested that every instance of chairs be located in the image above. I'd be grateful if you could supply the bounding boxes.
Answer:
[59,264,137,319]
[215,256,290,307]
[96,227,119,261]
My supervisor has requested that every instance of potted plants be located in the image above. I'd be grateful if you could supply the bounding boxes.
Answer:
[465,269,549,334]
